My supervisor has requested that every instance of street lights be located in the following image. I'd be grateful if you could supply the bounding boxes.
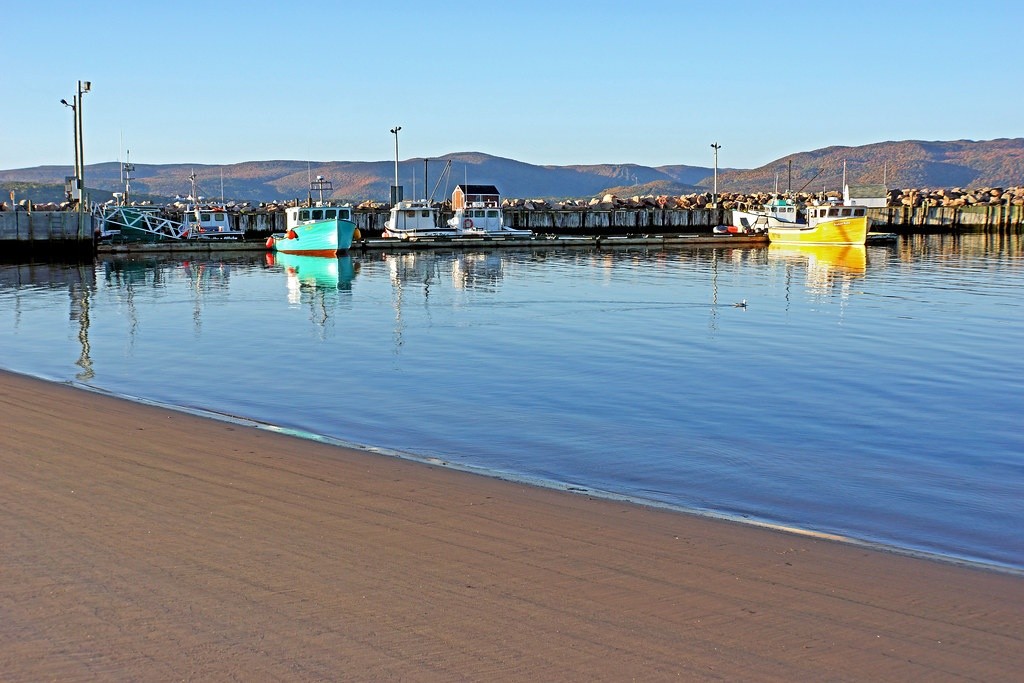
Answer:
[711,142,721,204]
[77,79,92,179]
[390,125,402,206]
[59,95,77,180]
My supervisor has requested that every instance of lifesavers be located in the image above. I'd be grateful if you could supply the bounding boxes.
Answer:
[462,218,473,229]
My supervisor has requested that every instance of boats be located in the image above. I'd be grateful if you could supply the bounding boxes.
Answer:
[767,242,868,276]
[731,198,798,232]
[766,197,874,230]
[382,198,457,240]
[266,251,360,326]
[767,215,873,245]
[267,175,357,253]
[446,199,533,237]
